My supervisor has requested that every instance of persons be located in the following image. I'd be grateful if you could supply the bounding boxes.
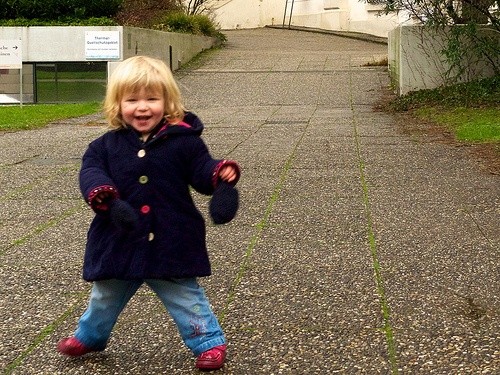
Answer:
[58,55,240,369]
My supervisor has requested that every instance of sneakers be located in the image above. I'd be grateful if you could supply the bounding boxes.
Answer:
[57,336,107,356]
[197,344,226,370]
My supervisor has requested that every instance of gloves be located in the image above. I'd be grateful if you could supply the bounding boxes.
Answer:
[107,198,141,235]
[210,182,239,224]
